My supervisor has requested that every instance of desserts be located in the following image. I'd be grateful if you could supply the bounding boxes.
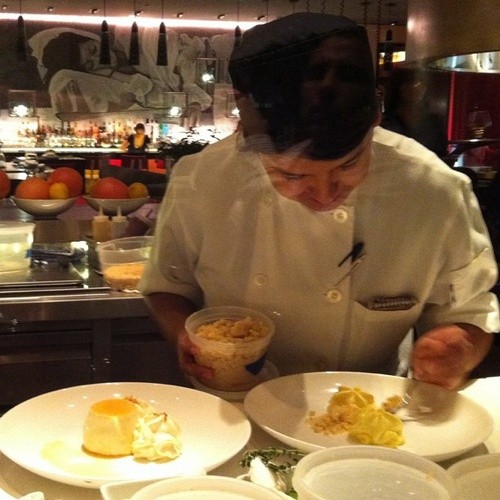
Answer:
[83,399,145,455]
[328,385,376,424]
[347,406,405,446]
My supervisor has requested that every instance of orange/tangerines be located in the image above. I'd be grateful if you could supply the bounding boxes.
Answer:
[127,182,148,199]
[50,183,68,199]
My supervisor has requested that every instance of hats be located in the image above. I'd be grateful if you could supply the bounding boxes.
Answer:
[227,12,377,162]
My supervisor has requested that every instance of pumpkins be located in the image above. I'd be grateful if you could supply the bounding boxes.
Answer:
[90,177,128,200]
[14,176,50,199]
[0,170,11,197]
[47,168,82,198]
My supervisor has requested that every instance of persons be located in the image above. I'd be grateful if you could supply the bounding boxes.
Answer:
[137,12,500,392]
[42,25,240,128]
[122,123,156,154]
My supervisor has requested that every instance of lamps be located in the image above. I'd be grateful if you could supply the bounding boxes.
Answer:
[201,66,215,83]
[419,0,500,75]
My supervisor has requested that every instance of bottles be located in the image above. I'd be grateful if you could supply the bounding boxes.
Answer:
[94,206,156,241]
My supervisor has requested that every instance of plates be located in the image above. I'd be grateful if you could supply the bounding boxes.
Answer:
[0,382,251,489]
[11,195,79,219]
[244,370,495,461]
[81,194,152,216]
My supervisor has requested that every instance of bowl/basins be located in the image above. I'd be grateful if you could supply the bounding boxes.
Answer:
[0,219,35,272]
[97,236,156,290]
[446,456,500,500]
[132,475,297,500]
[291,444,458,500]
[186,307,275,388]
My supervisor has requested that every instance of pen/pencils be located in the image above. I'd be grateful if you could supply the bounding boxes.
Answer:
[332,253,366,288]
[336,240,364,266]
